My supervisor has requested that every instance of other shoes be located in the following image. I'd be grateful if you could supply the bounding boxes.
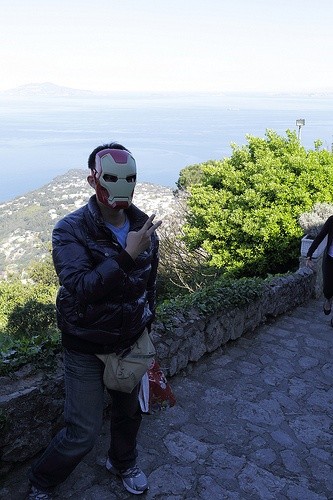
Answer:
[323,301,331,315]
[331,319,333,328]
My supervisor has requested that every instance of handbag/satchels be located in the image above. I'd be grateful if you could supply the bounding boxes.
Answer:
[96,328,156,394]
[138,359,175,413]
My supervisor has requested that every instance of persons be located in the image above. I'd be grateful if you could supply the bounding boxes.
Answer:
[304,215,333,328]
[28,142,162,500]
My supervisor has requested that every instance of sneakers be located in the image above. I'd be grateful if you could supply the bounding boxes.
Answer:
[25,468,49,500]
[105,456,149,495]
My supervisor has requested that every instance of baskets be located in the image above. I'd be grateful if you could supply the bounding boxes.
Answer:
[300,232,328,258]
